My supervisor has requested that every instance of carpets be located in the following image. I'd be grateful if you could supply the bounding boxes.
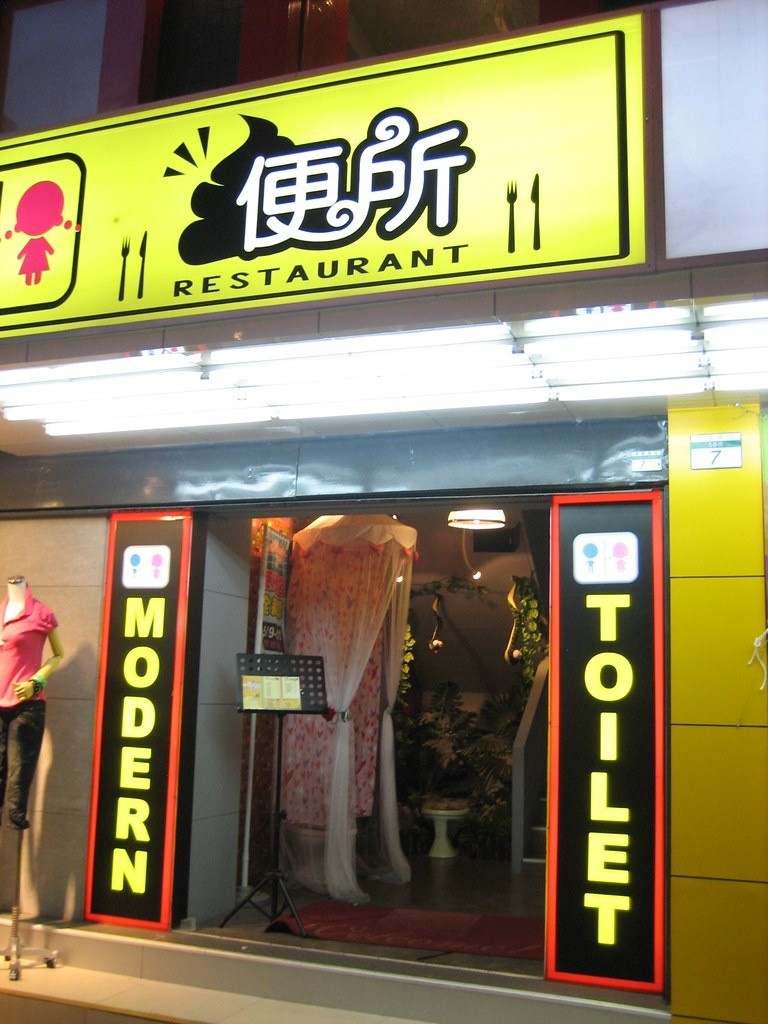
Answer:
[265,900,544,961]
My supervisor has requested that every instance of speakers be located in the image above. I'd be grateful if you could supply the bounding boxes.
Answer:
[473,520,521,553]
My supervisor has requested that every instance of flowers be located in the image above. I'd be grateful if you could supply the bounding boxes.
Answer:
[423,799,469,810]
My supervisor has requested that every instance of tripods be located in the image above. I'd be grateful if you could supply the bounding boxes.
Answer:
[217,654,328,940]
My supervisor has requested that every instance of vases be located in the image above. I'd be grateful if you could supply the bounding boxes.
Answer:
[422,809,470,858]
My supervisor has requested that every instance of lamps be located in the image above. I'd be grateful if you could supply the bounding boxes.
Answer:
[447,508,505,530]
